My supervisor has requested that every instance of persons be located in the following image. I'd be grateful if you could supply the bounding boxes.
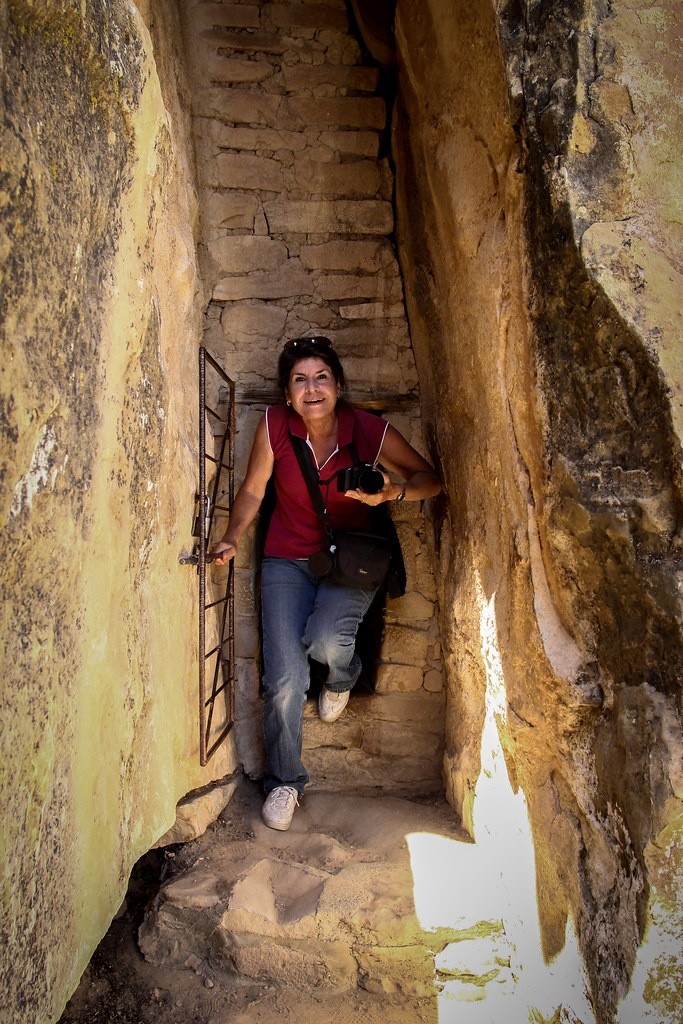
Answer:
[210,337,445,832]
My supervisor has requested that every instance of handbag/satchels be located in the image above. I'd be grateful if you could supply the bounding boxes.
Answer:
[309,527,391,593]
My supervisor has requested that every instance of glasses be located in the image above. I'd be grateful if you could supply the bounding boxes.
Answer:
[283,336,334,352]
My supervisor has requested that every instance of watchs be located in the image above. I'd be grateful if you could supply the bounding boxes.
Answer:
[395,482,406,501]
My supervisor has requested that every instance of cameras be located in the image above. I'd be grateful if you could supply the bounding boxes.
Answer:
[337,464,385,494]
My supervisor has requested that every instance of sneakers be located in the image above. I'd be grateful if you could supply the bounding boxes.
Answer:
[319,684,350,722]
[262,785,300,830]
